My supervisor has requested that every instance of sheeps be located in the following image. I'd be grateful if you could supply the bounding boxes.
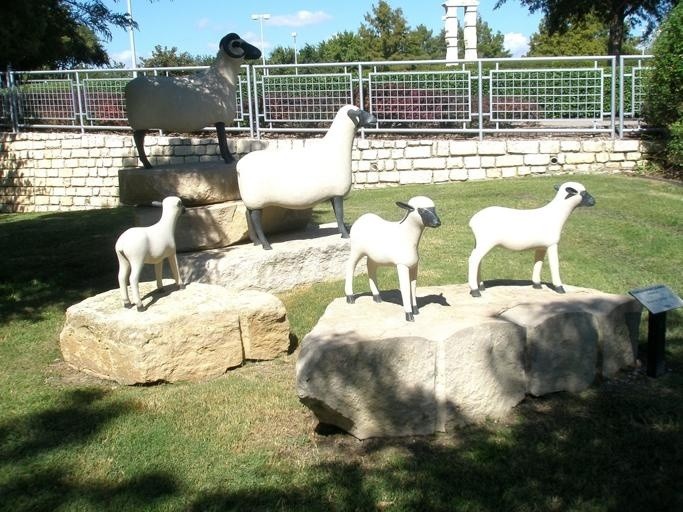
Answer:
[114,196,186,313]
[467,180,596,298]
[235,104,378,251]
[124,32,262,169]
[347,195,441,322]
